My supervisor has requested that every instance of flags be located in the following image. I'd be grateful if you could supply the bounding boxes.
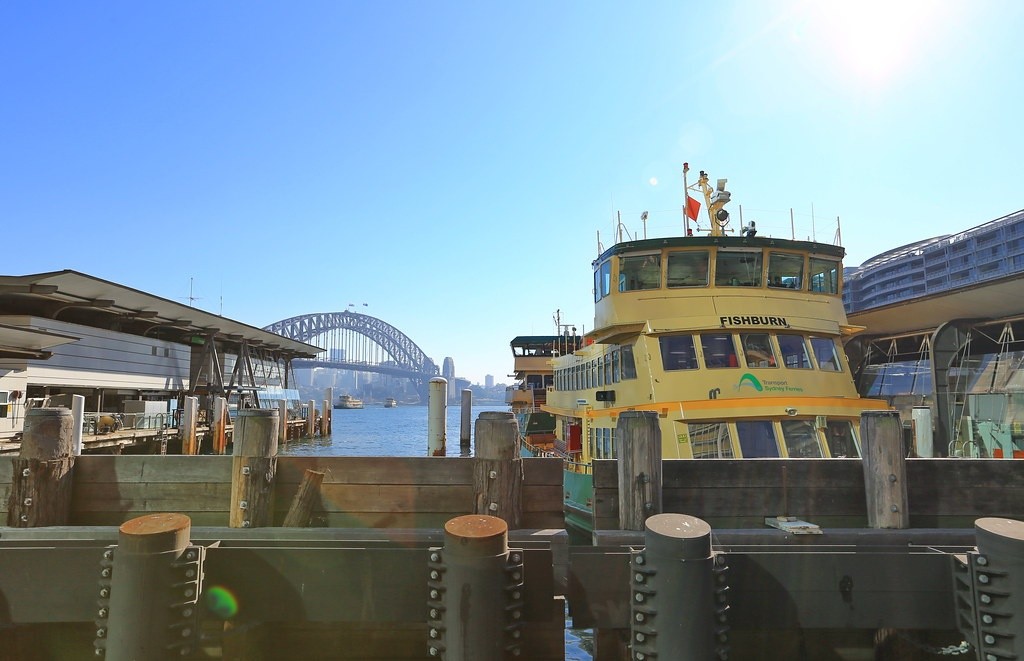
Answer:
[684,196,701,222]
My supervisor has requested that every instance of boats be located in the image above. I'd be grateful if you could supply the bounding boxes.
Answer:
[794,209,1023,462]
[503,162,901,476]
[384,397,397,409]
[333,394,364,409]
